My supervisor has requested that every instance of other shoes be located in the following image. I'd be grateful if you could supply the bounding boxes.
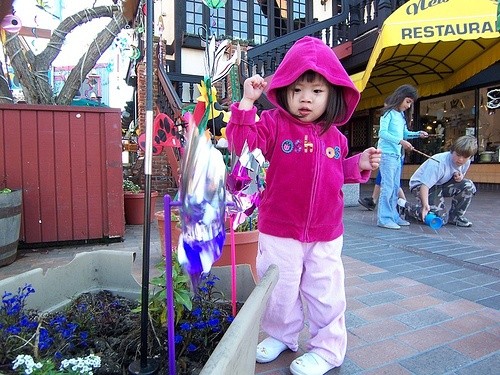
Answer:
[290,352,335,375]
[395,217,410,225]
[377,220,400,229]
[256,335,288,363]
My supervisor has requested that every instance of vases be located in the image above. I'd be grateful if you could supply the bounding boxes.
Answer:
[156,209,179,256]
[0,250,279,375]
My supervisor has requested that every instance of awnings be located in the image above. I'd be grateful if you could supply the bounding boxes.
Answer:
[346,0,500,114]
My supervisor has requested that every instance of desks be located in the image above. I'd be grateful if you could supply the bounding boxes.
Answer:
[370,162,500,192]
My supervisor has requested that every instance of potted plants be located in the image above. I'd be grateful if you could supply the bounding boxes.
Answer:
[172,213,258,285]
[0,188,23,267]
[122,177,158,225]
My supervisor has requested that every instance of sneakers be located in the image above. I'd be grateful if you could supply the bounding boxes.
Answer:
[397,198,407,220]
[449,216,472,227]
[358,197,376,211]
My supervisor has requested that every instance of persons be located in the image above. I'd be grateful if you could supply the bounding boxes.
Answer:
[376,84,428,230]
[225,35,382,375]
[358,95,407,211]
[397,136,476,227]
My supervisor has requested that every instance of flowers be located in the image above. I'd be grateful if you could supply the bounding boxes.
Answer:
[174,273,234,353]
[12,354,101,375]
[0,283,90,361]
